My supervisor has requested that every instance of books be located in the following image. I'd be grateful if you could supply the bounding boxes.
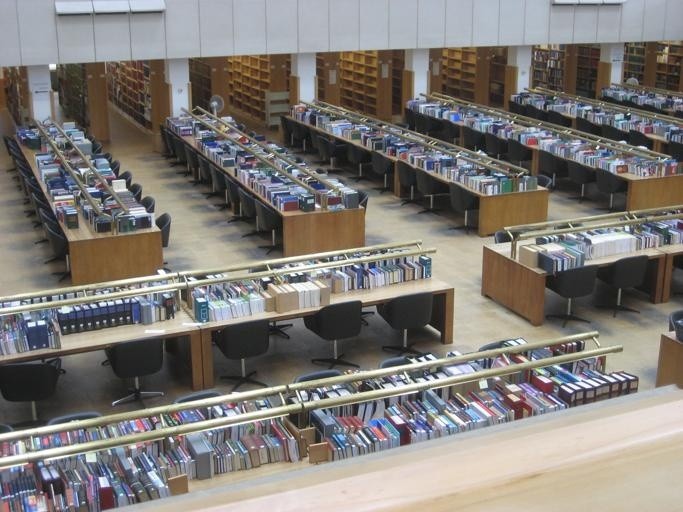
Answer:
[536,219,679,273]
[288,101,538,195]
[287,56,291,92]
[3,66,30,125]
[107,60,152,130]
[180,249,432,322]
[165,116,359,211]
[57,64,92,128]
[228,54,269,120]
[17,122,155,232]
[339,50,377,115]
[2,268,180,357]
[392,49,405,104]
[294,338,639,464]
[0,395,300,509]
[405,42,680,179]
[188,59,212,117]
[316,52,325,102]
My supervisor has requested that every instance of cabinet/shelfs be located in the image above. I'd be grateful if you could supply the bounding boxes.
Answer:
[0,41,682,142]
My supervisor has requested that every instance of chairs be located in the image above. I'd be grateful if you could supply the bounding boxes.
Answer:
[304,300,363,371]
[3,356,62,428]
[403,87,683,213]
[104,336,166,408]
[216,321,270,394]
[280,99,553,237]
[375,291,433,358]
[3,121,172,287]
[161,107,370,258]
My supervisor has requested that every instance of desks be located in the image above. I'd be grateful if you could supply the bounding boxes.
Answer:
[0,239,455,363]
[482,205,682,327]
[3,330,683,512]
